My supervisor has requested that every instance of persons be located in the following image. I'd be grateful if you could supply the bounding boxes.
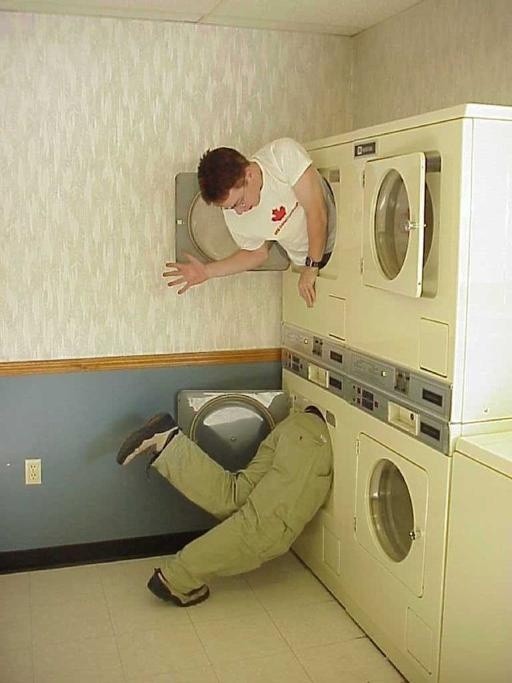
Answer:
[115,410,333,609]
[159,136,334,308]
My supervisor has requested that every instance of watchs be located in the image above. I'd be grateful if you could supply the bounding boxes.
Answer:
[305,256,324,270]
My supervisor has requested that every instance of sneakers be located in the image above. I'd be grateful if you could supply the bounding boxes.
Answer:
[147,567,210,608]
[116,411,183,467]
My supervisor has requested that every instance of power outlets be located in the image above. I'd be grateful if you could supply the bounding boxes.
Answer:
[24,457,42,484]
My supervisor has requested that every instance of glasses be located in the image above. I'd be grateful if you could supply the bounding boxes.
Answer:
[234,176,246,210]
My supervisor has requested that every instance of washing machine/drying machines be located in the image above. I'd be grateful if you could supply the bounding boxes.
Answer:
[174,102,511,683]
